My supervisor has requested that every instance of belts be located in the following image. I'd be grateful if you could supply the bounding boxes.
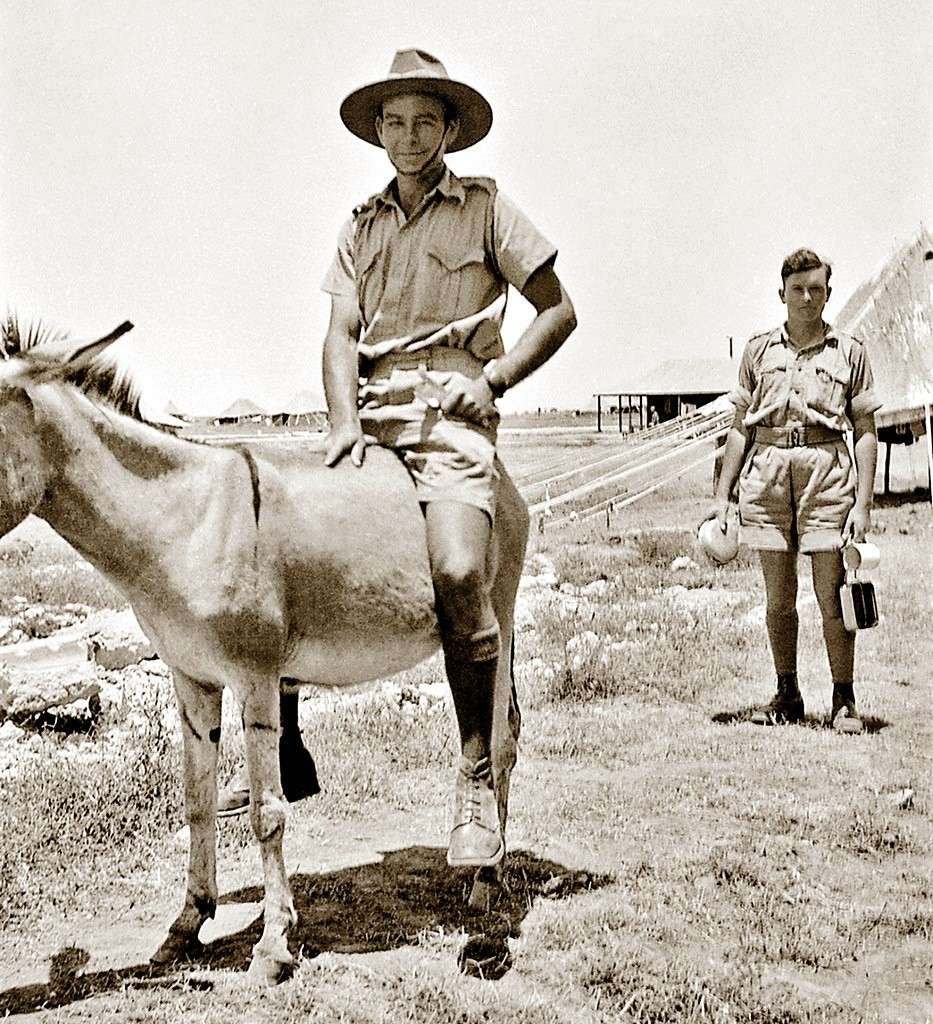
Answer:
[755,425,842,447]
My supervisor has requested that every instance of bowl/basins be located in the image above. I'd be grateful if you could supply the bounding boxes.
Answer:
[840,579,879,631]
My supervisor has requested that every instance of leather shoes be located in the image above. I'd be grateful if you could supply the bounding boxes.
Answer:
[827,700,862,735]
[448,770,506,865]
[213,747,320,819]
[746,700,804,726]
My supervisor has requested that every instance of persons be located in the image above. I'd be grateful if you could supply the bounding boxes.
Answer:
[190,47,575,872]
[692,249,883,735]
[648,406,659,428]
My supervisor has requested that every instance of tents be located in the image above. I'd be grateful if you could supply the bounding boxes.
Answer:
[505,223,932,541]
[141,389,327,435]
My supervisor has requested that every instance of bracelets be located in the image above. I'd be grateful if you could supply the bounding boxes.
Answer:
[484,368,507,397]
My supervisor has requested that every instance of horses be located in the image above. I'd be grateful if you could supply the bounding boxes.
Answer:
[1,307,531,986]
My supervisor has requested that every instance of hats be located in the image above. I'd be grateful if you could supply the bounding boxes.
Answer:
[338,46,494,153]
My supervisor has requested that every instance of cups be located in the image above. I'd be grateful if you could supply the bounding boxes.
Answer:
[843,534,881,570]
